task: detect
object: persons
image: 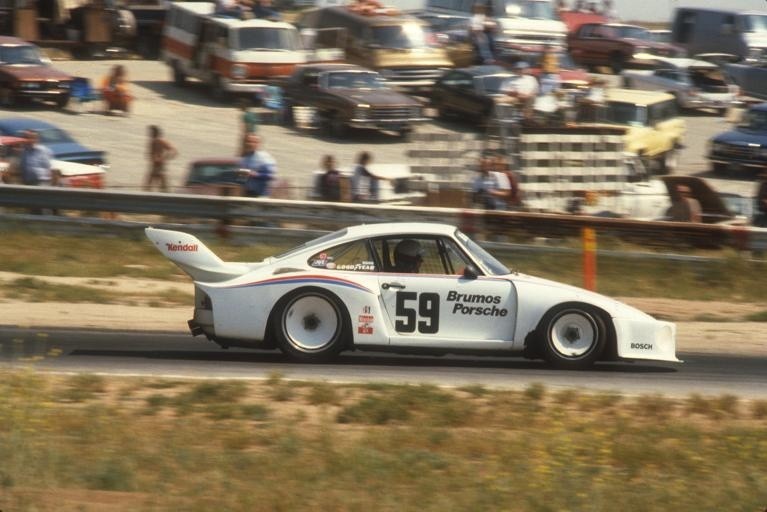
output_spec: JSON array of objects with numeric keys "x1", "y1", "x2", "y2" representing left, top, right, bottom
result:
[
  {"x1": 499, "y1": 55, "x2": 566, "y2": 135},
  {"x1": 113, "y1": 4, "x2": 141, "y2": 61},
  {"x1": 468, "y1": 1, "x2": 497, "y2": 66},
  {"x1": 314, "y1": 152, "x2": 391, "y2": 204},
  {"x1": 660, "y1": 152, "x2": 678, "y2": 196},
  {"x1": 555, "y1": 0, "x2": 617, "y2": 22},
  {"x1": 235, "y1": 107, "x2": 279, "y2": 197},
  {"x1": 392, "y1": 240, "x2": 425, "y2": 272},
  {"x1": 17, "y1": 130, "x2": 62, "y2": 219},
  {"x1": 757, "y1": 181, "x2": 767, "y2": 213},
  {"x1": 146, "y1": 125, "x2": 178, "y2": 191},
  {"x1": 103, "y1": 66, "x2": 131, "y2": 117},
  {"x1": 671, "y1": 186, "x2": 703, "y2": 222},
  {"x1": 473, "y1": 155, "x2": 523, "y2": 212}
]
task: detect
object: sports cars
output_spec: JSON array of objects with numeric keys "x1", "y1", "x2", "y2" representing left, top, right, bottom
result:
[{"x1": 144, "y1": 221, "x2": 684, "y2": 369}]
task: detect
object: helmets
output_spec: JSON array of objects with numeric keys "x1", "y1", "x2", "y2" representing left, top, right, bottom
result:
[{"x1": 393, "y1": 238, "x2": 424, "y2": 265}]
